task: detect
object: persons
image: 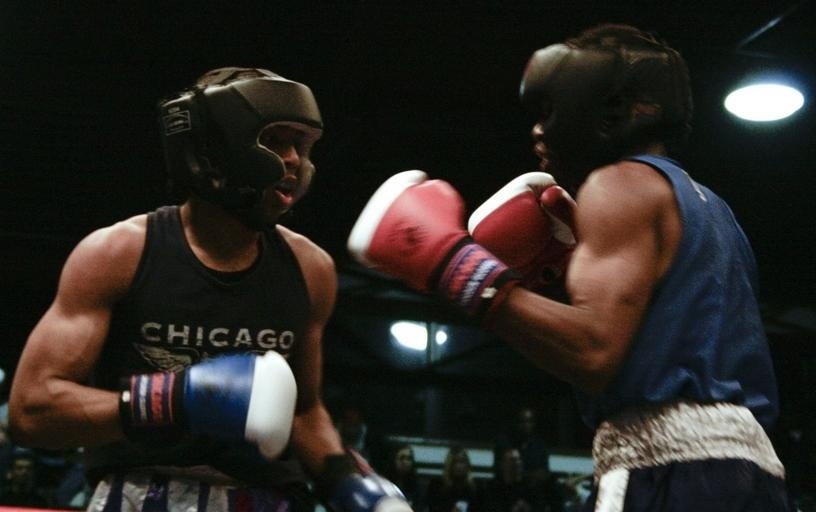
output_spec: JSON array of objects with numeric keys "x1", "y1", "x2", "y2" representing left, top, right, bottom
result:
[
  {"x1": 7, "y1": 65, "x2": 413, "y2": 512},
  {"x1": 345, "y1": 22, "x2": 791, "y2": 512}
]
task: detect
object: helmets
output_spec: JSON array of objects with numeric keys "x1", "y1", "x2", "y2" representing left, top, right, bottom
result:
[
  {"x1": 513, "y1": 22, "x2": 698, "y2": 192},
  {"x1": 152, "y1": 67, "x2": 324, "y2": 208}
]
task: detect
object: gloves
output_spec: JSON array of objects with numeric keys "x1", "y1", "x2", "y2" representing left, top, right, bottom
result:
[
  {"x1": 115, "y1": 348, "x2": 300, "y2": 469},
  {"x1": 347, "y1": 165, "x2": 523, "y2": 324},
  {"x1": 462, "y1": 170, "x2": 581, "y2": 295},
  {"x1": 311, "y1": 449, "x2": 416, "y2": 511}
]
